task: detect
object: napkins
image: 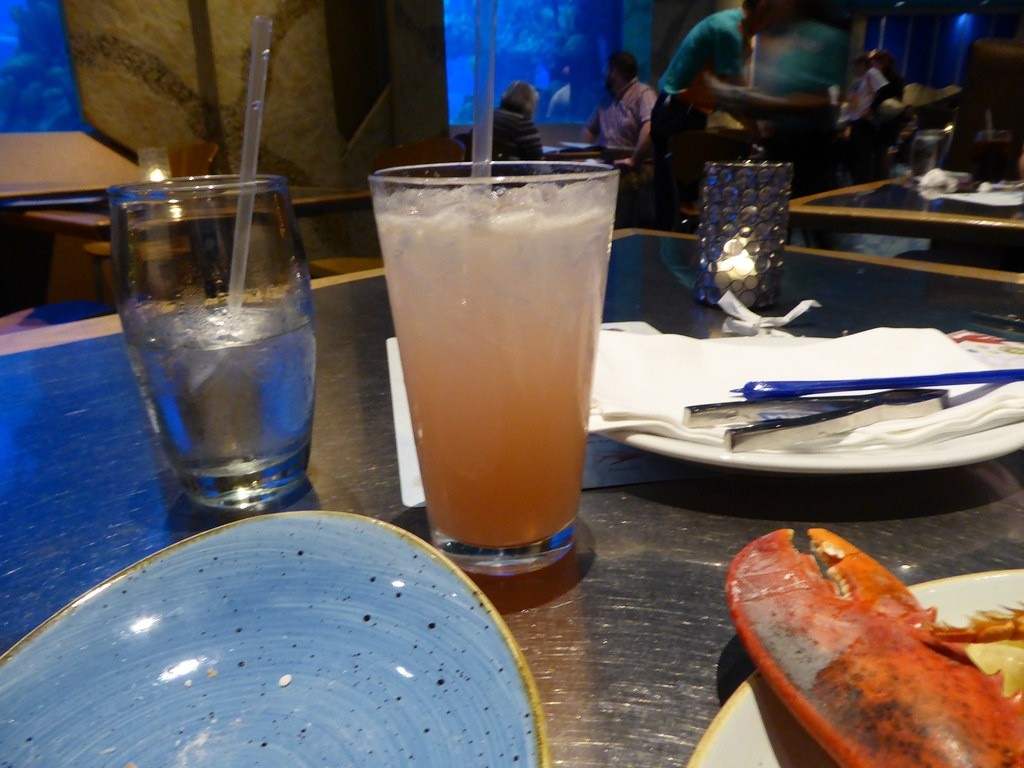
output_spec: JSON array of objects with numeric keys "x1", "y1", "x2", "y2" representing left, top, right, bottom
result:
[{"x1": 589, "y1": 326, "x2": 1024, "y2": 452}]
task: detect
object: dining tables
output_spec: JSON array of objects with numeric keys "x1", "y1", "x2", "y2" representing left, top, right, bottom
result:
[
  {"x1": 1, "y1": 235, "x2": 1024, "y2": 768},
  {"x1": 787, "y1": 180, "x2": 1024, "y2": 245},
  {"x1": 26, "y1": 191, "x2": 372, "y2": 312}
]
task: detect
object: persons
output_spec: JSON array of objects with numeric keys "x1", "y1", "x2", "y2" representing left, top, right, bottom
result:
[
  {"x1": 580, "y1": 53, "x2": 659, "y2": 193},
  {"x1": 462, "y1": 80, "x2": 543, "y2": 189},
  {"x1": 753, "y1": 0, "x2": 852, "y2": 251},
  {"x1": 650, "y1": 0, "x2": 805, "y2": 232},
  {"x1": 832, "y1": 46, "x2": 919, "y2": 186}
]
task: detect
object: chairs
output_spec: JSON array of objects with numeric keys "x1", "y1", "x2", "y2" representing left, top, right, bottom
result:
[{"x1": 84, "y1": 142, "x2": 222, "y2": 307}]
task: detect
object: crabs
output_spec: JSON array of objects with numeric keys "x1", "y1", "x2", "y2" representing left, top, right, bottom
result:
[{"x1": 724, "y1": 526, "x2": 1024, "y2": 768}]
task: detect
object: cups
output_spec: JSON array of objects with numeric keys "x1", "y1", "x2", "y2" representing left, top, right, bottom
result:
[
  {"x1": 694, "y1": 159, "x2": 792, "y2": 312},
  {"x1": 106, "y1": 172, "x2": 318, "y2": 517},
  {"x1": 910, "y1": 128, "x2": 952, "y2": 185},
  {"x1": 369, "y1": 159, "x2": 620, "y2": 577}
]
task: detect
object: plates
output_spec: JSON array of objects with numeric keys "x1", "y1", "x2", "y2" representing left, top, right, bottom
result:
[
  {"x1": 596, "y1": 421, "x2": 1024, "y2": 475},
  {"x1": 0, "y1": 510, "x2": 550, "y2": 768},
  {"x1": 685, "y1": 569, "x2": 1022, "y2": 766}
]
